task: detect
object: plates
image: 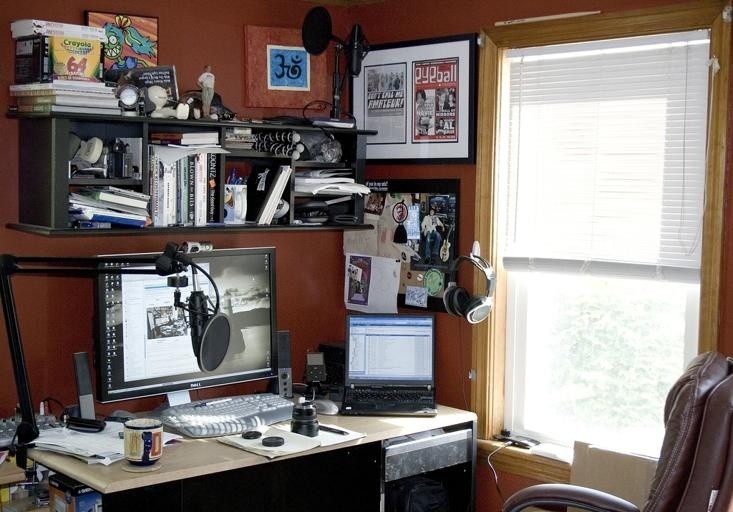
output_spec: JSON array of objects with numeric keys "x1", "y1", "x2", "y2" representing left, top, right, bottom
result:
[{"x1": 424, "y1": 269, "x2": 443, "y2": 297}]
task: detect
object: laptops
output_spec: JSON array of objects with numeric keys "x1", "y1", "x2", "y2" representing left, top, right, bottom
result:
[{"x1": 341, "y1": 314, "x2": 437, "y2": 416}]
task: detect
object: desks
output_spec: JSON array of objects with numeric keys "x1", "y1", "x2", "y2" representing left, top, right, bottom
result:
[{"x1": 25, "y1": 397, "x2": 481, "y2": 510}]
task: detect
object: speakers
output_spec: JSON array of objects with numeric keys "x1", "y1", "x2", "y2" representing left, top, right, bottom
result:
[
  {"x1": 73, "y1": 352, "x2": 96, "y2": 420},
  {"x1": 271, "y1": 330, "x2": 293, "y2": 398}
]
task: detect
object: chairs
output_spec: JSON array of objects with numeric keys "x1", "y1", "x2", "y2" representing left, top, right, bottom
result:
[{"x1": 502, "y1": 345, "x2": 733, "y2": 512}]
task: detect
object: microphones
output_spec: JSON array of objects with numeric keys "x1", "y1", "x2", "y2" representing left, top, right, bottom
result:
[
  {"x1": 188, "y1": 291, "x2": 208, "y2": 357},
  {"x1": 347, "y1": 24, "x2": 364, "y2": 76}
]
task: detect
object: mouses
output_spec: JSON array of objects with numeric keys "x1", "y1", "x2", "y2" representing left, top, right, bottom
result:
[{"x1": 311, "y1": 400, "x2": 339, "y2": 415}]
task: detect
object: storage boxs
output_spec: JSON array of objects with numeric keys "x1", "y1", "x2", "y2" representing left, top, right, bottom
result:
[{"x1": 13, "y1": 36, "x2": 106, "y2": 84}]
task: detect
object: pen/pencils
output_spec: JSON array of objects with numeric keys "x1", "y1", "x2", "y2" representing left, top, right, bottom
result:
[
  {"x1": 106, "y1": 417, "x2": 129, "y2": 422},
  {"x1": 319, "y1": 425, "x2": 348, "y2": 435},
  {"x1": 193, "y1": 397, "x2": 232, "y2": 409}
]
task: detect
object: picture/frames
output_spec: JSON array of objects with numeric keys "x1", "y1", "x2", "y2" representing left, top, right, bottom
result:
[
  {"x1": 86, "y1": 10, "x2": 160, "y2": 80},
  {"x1": 349, "y1": 31, "x2": 479, "y2": 165}
]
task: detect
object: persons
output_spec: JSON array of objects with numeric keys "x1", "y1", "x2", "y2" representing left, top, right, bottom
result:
[
  {"x1": 387, "y1": 71, "x2": 404, "y2": 90},
  {"x1": 420, "y1": 208, "x2": 445, "y2": 265},
  {"x1": 415, "y1": 87, "x2": 456, "y2": 136},
  {"x1": 366, "y1": 195, "x2": 384, "y2": 211},
  {"x1": 395, "y1": 205, "x2": 405, "y2": 220},
  {"x1": 196, "y1": 64, "x2": 215, "y2": 118}
]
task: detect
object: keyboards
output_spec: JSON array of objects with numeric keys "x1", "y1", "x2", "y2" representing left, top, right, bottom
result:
[{"x1": 159, "y1": 393, "x2": 295, "y2": 437}]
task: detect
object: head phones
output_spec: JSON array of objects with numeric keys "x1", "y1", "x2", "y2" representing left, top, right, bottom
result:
[{"x1": 443, "y1": 255, "x2": 496, "y2": 324}]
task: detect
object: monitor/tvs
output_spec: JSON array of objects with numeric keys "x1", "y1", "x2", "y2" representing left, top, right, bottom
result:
[{"x1": 92, "y1": 247, "x2": 279, "y2": 407}]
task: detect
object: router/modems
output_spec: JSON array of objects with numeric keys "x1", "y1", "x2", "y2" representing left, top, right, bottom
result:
[{"x1": 501, "y1": 435, "x2": 540, "y2": 449}]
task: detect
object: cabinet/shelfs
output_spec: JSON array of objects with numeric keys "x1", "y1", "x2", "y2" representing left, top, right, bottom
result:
[{"x1": 5, "y1": 104, "x2": 378, "y2": 240}]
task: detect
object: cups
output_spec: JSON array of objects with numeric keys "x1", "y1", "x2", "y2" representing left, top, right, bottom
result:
[
  {"x1": 225, "y1": 185, "x2": 247, "y2": 225},
  {"x1": 124, "y1": 418, "x2": 163, "y2": 466}
]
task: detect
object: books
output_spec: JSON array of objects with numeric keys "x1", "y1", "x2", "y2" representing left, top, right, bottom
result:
[
  {"x1": 9, "y1": 19, "x2": 123, "y2": 116},
  {"x1": 245, "y1": 164, "x2": 293, "y2": 226},
  {"x1": 67, "y1": 130, "x2": 222, "y2": 230}
]
